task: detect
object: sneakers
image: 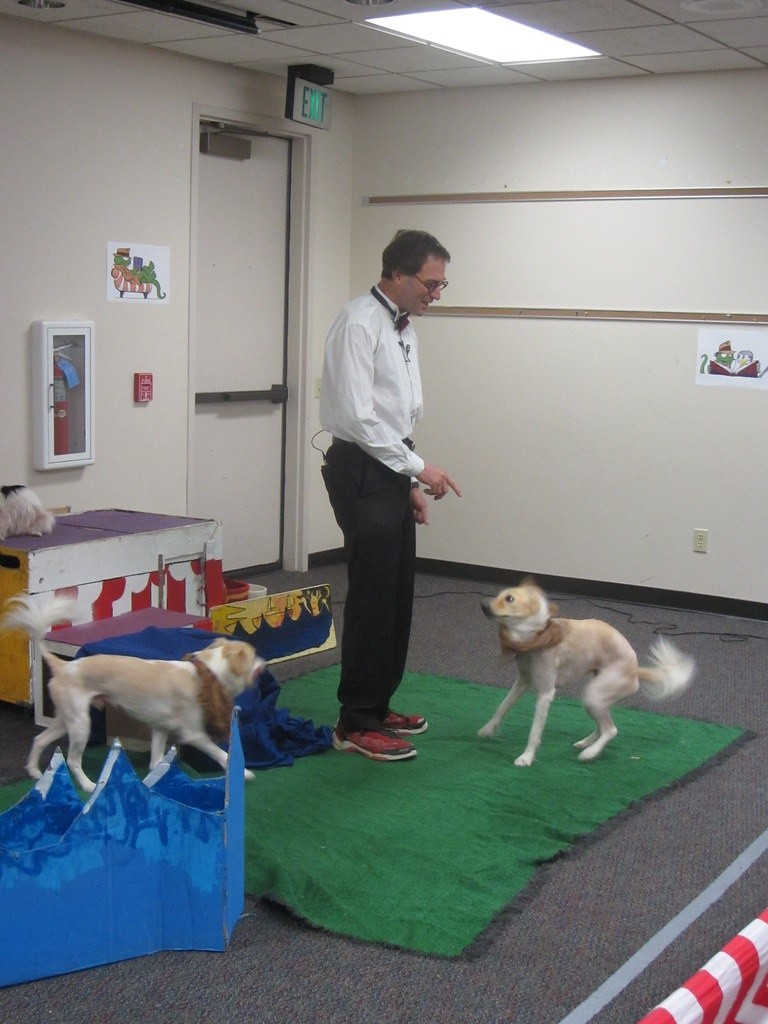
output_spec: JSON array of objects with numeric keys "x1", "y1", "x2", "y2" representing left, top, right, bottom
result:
[
  {"x1": 382, "y1": 708, "x2": 428, "y2": 736},
  {"x1": 332, "y1": 713, "x2": 417, "y2": 761}
]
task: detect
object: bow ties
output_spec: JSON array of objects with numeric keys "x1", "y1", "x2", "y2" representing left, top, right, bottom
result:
[{"x1": 370, "y1": 285, "x2": 411, "y2": 332}]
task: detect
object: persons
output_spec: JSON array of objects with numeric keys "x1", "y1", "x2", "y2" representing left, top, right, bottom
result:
[{"x1": 319, "y1": 229, "x2": 461, "y2": 759}]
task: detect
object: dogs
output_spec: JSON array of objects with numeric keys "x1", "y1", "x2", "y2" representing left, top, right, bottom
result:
[
  {"x1": 0, "y1": 485, "x2": 56, "y2": 542},
  {"x1": 5, "y1": 594, "x2": 267, "y2": 792},
  {"x1": 478, "y1": 581, "x2": 698, "y2": 767}
]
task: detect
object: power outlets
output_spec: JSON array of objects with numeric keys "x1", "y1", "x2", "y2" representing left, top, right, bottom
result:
[{"x1": 693, "y1": 528, "x2": 710, "y2": 554}]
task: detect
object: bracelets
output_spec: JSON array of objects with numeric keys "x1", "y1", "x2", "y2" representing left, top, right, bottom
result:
[{"x1": 411, "y1": 482, "x2": 419, "y2": 489}]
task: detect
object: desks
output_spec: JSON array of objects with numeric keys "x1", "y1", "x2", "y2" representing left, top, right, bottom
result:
[{"x1": 0, "y1": 508, "x2": 221, "y2": 707}]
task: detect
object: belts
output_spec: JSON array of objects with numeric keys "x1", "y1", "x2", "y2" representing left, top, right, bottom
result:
[{"x1": 331, "y1": 434, "x2": 417, "y2": 454}]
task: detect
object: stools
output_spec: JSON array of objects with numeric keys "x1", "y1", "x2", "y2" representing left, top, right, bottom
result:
[{"x1": 30, "y1": 607, "x2": 212, "y2": 728}]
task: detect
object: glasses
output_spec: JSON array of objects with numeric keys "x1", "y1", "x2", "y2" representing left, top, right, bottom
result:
[{"x1": 413, "y1": 271, "x2": 449, "y2": 291}]
